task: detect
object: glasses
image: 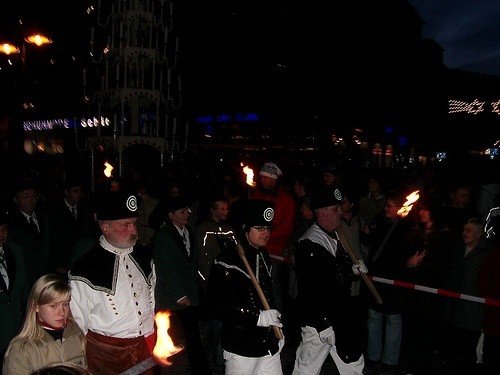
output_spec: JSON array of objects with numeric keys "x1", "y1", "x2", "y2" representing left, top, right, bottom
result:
[{"x1": 251, "y1": 226, "x2": 272, "y2": 232}]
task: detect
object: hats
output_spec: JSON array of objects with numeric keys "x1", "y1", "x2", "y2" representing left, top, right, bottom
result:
[
  {"x1": 259, "y1": 163, "x2": 282, "y2": 180},
  {"x1": 310, "y1": 188, "x2": 343, "y2": 210},
  {"x1": 97, "y1": 193, "x2": 143, "y2": 220},
  {"x1": 351, "y1": 259, "x2": 369, "y2": 275},
  {"x1": 245, "y1": 204, "x2": 274, "y2": 226}
]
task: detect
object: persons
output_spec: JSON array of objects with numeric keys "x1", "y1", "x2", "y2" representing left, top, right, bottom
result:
[
  {"x1": 0, "y1": 272, "x2": 88, "y2": 375},
  {"x1": 0, "y1": 140, "x2": 500, "y2": 375},
  {"x1": 30, "y1": 360, "x2": 95, "y2": 375},
  {"x1": 205, "y1": 196, "x2": 293, "y2": 375},
  {"x1": 61, "y1": 188, "x2": 166, "y2": 374},
  {"x1": 282, "y1": 178, "x2": 373, "y2": 375}
]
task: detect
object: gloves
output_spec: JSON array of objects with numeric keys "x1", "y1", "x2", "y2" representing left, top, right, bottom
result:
[
  {"x1": 319, "y1": 326, "x2": 336, "y2": 346},
  {"x1": 257, "y1": 309, "x2": 283, "y2": 328}
]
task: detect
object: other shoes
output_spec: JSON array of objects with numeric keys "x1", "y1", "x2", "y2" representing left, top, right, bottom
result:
[
  {"x1": 365, "y1": 358, "x2": 380, "y2": 367},
  {"x1": 380, "y1": 363, "x2": 399, "y2": 372}
]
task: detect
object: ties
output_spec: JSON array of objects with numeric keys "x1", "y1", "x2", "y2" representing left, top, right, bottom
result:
[
  {"x1": 29, "y1": 217, "x2": 38, "y2": 232},
  {"x1": 72, "y1": 208, "x2": 75, "y2": 215}
]
